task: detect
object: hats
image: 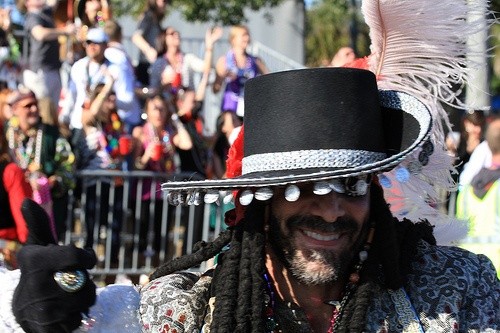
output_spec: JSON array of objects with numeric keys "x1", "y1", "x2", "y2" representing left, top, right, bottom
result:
[
  {"x1": 161, "y1": 0, "x2": 499, "y2": 206},
  {"x1": 7, "y1": 88, "x2": 34, "y2": 104},
  {"x1": 86, "y1": 28, "x2": 108, "y2": 43}
]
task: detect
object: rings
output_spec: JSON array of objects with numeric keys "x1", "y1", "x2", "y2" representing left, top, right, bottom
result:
[{"x1": 52, "y1": 270, "x2": 84, "y2": 305}]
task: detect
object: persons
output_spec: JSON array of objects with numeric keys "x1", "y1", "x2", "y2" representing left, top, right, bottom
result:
[
  {"x1": 0, "y1": 0, "x2": 500, "y2": 288},
  {"x1": 12, "y1": 67, "x2": 500, "y2": 333}
]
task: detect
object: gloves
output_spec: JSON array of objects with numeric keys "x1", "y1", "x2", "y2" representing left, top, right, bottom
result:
[{"x1": 12, "y1": 199, "x2": 97, "y2": 333}]
